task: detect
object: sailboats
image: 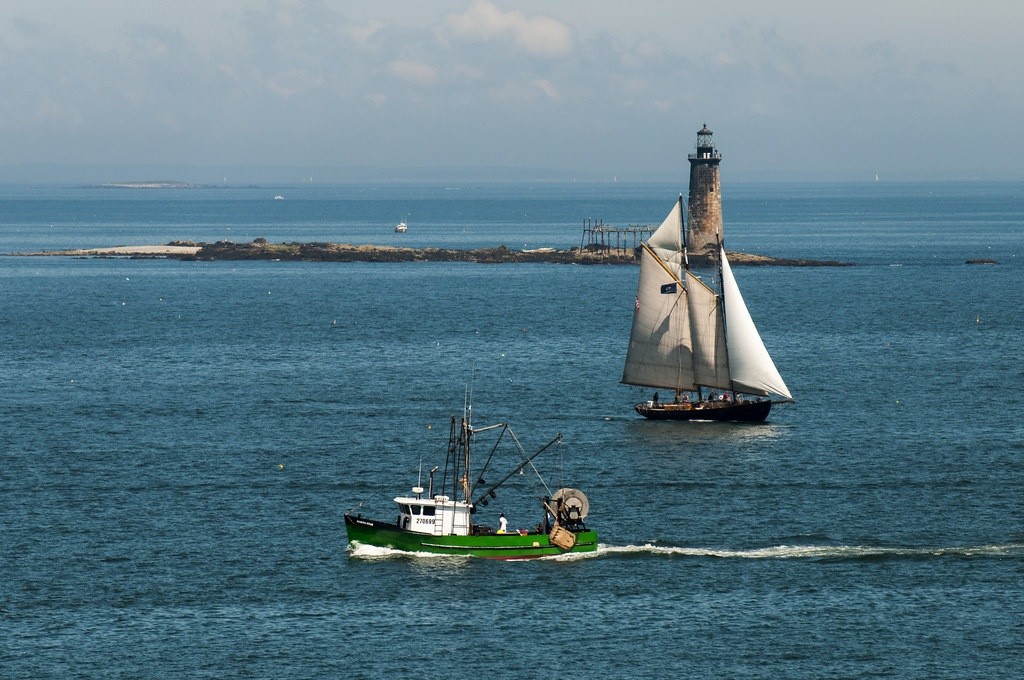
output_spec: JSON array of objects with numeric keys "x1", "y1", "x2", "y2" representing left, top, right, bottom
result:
[{"x1": 618, "y1": 193, "x2": 797, "y2": 425}]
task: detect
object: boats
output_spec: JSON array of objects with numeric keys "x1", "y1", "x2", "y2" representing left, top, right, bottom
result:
[
  {"x1": 395, "y1": 223, "x2": 408, "y2": 234},
  {"x1": 345, "y1": 381, "x2": 600, "y2": 557}
]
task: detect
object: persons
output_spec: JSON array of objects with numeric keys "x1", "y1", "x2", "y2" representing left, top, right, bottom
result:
[
  {"x1": 499, "y1": 513, "x2": 508, "y2": 533},
  {"x1": 653, "y1": 391, "x2": 659, "y2": 405},
  {"x1": 683, "y1": 394, "x2": 687, "y2": 401},
  {"x1": 708, "y1": 392, "x2": 714, "y2": 402},
  {"x1": 723, "y1": 391, "x2": 729, "y2": 399},
  {"x1": 674, "y1": 397, "x2": 679, "y2": 404}
]
